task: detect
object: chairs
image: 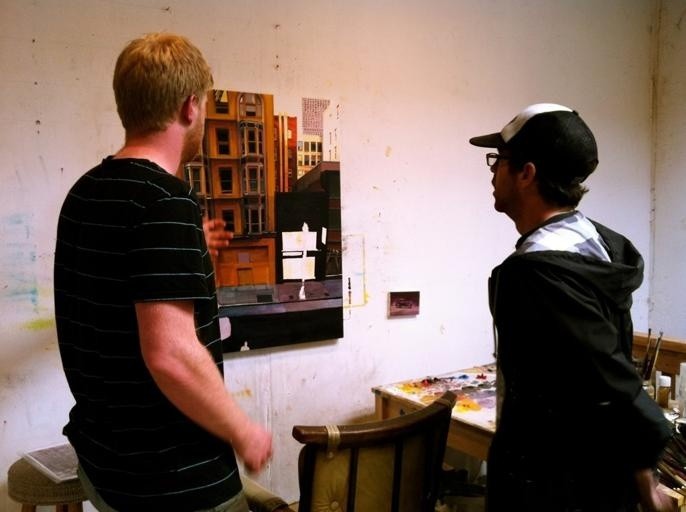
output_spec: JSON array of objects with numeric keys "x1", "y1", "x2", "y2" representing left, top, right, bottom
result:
[{"x1": 240, "y1": 389, "x2": 458, "y2": 511}]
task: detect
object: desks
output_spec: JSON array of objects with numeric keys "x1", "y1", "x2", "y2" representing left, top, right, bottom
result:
[{"x1": 369, "y1": 330, "x2": 686, "y2": 507}]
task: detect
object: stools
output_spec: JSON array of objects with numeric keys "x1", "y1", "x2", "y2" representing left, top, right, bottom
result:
[{"x1": 6, "y1": 443, "x2": 91, "y2": 511}]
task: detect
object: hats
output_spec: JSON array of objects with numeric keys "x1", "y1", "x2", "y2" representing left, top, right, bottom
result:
[{"x1": 469, "y1": 103, "x2": 598, "y2": 185}]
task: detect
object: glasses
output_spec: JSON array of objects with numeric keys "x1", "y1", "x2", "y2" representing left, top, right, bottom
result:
[{"x1": 486, "y1": 152, "x2": 514, "y2": 166}]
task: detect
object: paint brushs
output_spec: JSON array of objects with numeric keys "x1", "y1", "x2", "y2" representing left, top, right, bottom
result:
[
  {"x1": 656, "y1": 431, "x2": 686, "y2": 497},
  {"x1": 639, "y1": 328, "x2": 663, "y2": 380}
]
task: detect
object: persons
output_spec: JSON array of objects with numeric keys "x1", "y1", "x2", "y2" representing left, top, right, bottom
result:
[
  {"x1": 470, "y1": 103, "x2": 675, "y2": 512},
  {"x1": 55, "y1": 34, "x2": 276, "y2": 511}
]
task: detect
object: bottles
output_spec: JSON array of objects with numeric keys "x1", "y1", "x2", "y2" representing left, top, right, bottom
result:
[
  {"x1": 657, "y1": 376, "x2": 672, "y2": 410},
  {"x1": 642, "y1": 380, "x2": 654, "y2": 400},
  {"x1": 679, "y1": 362, "x2": 686, "y2": 418}
]
